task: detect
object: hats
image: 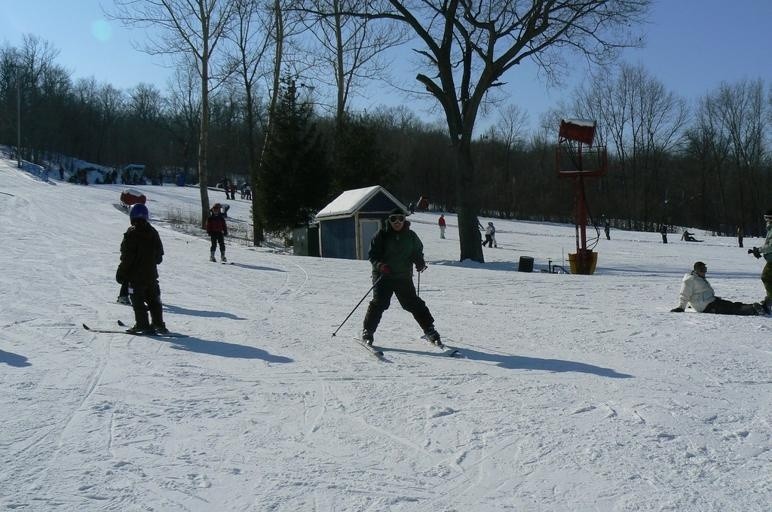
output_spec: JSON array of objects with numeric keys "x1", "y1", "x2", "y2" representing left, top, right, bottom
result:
[
  {"x1": 694, "y1": 261, "x2": 706, "y2": 271},
  {"x1": 389, "y1": 207, "x2": 404, "y2": 215}
]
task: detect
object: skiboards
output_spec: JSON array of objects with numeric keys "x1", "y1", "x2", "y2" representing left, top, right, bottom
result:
[
  {"x1": 82, "y1": 321, "x2": 191, "y2": 337},
  {"x1": 107, "y1": 297, "x2": 151, "y2": 313},
  {"x1": 353, "y1": 333, "x2": 459, "y2": 357},
  {"x1": 209, "y1": 259, "x2": 234, "y2": 265}
]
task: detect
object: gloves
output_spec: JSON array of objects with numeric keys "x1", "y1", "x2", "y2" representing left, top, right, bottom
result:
[
  {"x1": 670, "y1": 305, "x2": 692, "y2": 313},
  {"x1": 748, "y1": 246, "x2": 762, "y2": 259},
  {"x1": 377, "y1": 263, "x2": 390, "y2": 275},
  {"x1": 416, "y1": 263, "x2": 428, "y2": 273}
]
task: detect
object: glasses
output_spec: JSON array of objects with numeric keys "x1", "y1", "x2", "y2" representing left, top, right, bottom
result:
[
  {"x1": 389, "y1": 216, "x2": 405, "y2": 224},
  {"x1": 764, "y1": 215, "x2": 772, "y2": 221}
]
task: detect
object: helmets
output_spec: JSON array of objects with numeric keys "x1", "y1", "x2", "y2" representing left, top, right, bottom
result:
[{"x1": 130, "y1": 203, "x2": 149, "y2": 220}]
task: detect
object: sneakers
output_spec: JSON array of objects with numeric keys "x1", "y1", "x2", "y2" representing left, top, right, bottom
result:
[
  {"x1": 426, "y1": 331, "x2": 441, "y2": 346},
  {"x1": 126, "y1": 321, "x2": 166, "y2": 332},
  {"x1": 753, "y1": 295, "x2": 772, "y2": 315},
  {"x1": 210, "y1": 256, "x2": 227, "y2": 262},
  {"x1": 361, "y1": 330, "x2": 375, "y2": 346},
  {"x1": 117, "y1": 296, "x2": 130, "y2": 305}
]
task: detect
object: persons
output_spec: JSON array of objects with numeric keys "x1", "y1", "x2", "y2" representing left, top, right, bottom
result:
[
  {"x1": 489, "y1": 222, "x2": 497, "y2": 247},
  {"x1": 733, "y1": 223, "x2": 743, "y2": 247},
  {"x1": 56, "y1": 164, "x2": 252, "y2": 201},
  {"x1": 120, "y1": 203, "x2": 169, "y2": 334},
  {"x1": 438, "y1": 213, "x2": 446, "y2": 240},
  {"x1": 116, "y1": 279, "x2": 149, "y2": 311},
  {"x1": 206, "y1": 205, "x2": 230, "y2": 263},
  {"x1": 481, "y1": 222, "x2": 492, "y2": 248},
  {"x1": 602, "y1": 217, "x2": 610, "y2": 240},
  {"x1": 679, "y1": 230, "x2": 696, "y2": 241},
  {"x1": 747, "y1": 208, "x2": 771, "y2": 314},
  {"x1": 358, "y1": 208, "x2": 442, "y2": 348},
  {"x1": 659, "y1": 225, "x2": 667, "y2": 243},
  {"x1": 669, "y1": 262, "x2": 769, "y2": 316}
]
